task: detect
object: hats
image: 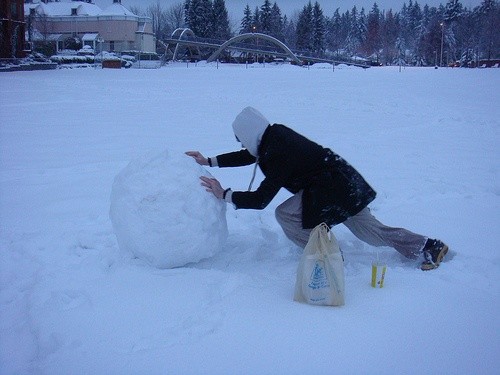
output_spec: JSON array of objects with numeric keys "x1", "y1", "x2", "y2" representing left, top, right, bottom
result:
[{"x1": 231, "y1": 106, "x2": 270, "y2": 158}]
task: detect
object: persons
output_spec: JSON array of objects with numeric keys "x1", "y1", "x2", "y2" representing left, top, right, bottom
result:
[{"x1": 186, "y1": 107, "x2": 448, "y2": 270}]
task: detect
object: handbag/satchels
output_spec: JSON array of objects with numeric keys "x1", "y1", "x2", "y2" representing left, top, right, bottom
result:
[{"x1": 292, "y1": 222, "x2": 346, "y2": 306}]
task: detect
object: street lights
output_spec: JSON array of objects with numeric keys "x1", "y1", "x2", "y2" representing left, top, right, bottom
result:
[
  {"x1": 440, "y1": 23, "x2": 444, "y2": 67},
  {"x1": 253, "y1": 26, "x2": 259, "y2": 62}
]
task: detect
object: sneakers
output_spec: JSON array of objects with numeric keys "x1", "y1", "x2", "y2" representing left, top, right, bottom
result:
[{"x1": 420, "y1": 239, "x2": 449, "y2": 270}]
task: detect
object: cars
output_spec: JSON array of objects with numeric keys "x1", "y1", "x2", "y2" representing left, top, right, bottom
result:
[{"x1": 179, "y1": 55, "x2": 201, "y2": 63}]
